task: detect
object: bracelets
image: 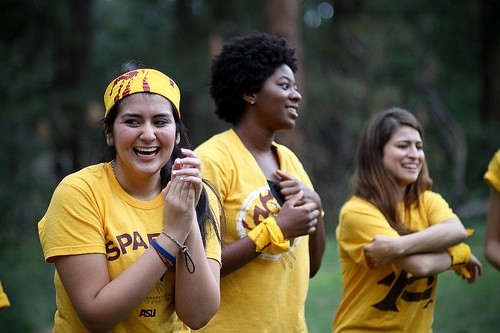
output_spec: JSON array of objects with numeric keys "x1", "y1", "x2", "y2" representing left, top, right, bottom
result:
[
  {"x1": 149, "y1": 238, "x2": 176, "y2": 270},
  {"x1": 161, "y1": 231, "x2": 195, "y2": 274}
]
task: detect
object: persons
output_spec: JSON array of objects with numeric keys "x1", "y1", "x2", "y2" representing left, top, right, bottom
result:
[
  {"x1": 37, "y1": 69, "x2": 227, "y2": 333},
  {"x1": 192, "y1": 31, "x2": 327, "y2": 333},
  {"x1": 335, "y1": 106, "x2": 482, "y2": 333},
  {"x1": 483, "y1": 148, "x2": 500, "y2": 272}
]
